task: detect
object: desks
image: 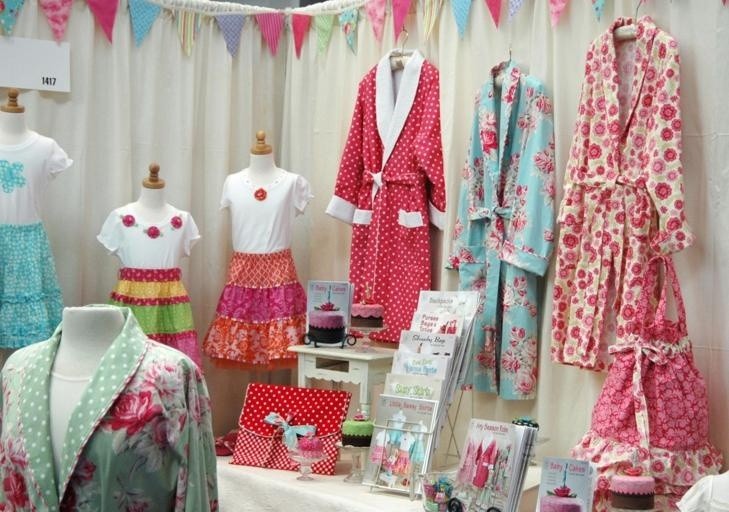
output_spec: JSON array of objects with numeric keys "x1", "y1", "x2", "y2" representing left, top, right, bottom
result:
[
  {"x1": 289, "y1": 344, "x2": 396, "y2": 419},
  {"x1": 216, "y1": 445, "x2": 543, "y2": 511}
]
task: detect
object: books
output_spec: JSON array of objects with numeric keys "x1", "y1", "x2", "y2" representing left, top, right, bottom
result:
[
  {"x1": 362, "y1": 288, "x2": 486, "y2": 498},
  {"x1": 453, "y1": 416, "x2": 537, "y2": 512},
  {"x1": 536, "y1": 455, "x2": 598, "y2": 512},
  {"x1": 305, "y1": 279, "x2": 353, "y2": 336}
]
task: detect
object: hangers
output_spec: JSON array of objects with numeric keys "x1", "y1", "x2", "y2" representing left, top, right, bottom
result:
[
  {"x1": 493, "y1": 42, "x2": 511, "y2": 89},
  {"x1": 388, "y1": 27, "x2": 409, "y2": 72},
  {"x1": 612, "y1": 0, "x2": 642, "y2": 42}
]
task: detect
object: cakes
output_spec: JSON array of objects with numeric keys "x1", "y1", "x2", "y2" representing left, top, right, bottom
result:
[
  {"x1": 341, "y1": 419, "x2": 375, "y2": 448},
  {"x1": 308, "y1": 310, "x2": 345, "y2": 344},
  {"x1": 349, "y1": 302, "x2": 385, "y2": 326},
  {"x1": 539, "y1": 493, "x2": 581, "y2": 511},
  {"x1": 610, "y1": 474, "x2": 656, "y2": 510}
]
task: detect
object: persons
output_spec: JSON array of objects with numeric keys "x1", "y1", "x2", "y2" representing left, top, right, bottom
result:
[
  {"x1": 93, "y1": 177, "x2": 207, "y2": 381},
  {"x1": 201, "y1": 145, "x2": 315, "y2": 370},
  {"x1": 1, "y1": 303, "x2": 220, "y2": 512},
  {"x1": 1, "y1": 104, "x2": 76, "y2": 352}
]
